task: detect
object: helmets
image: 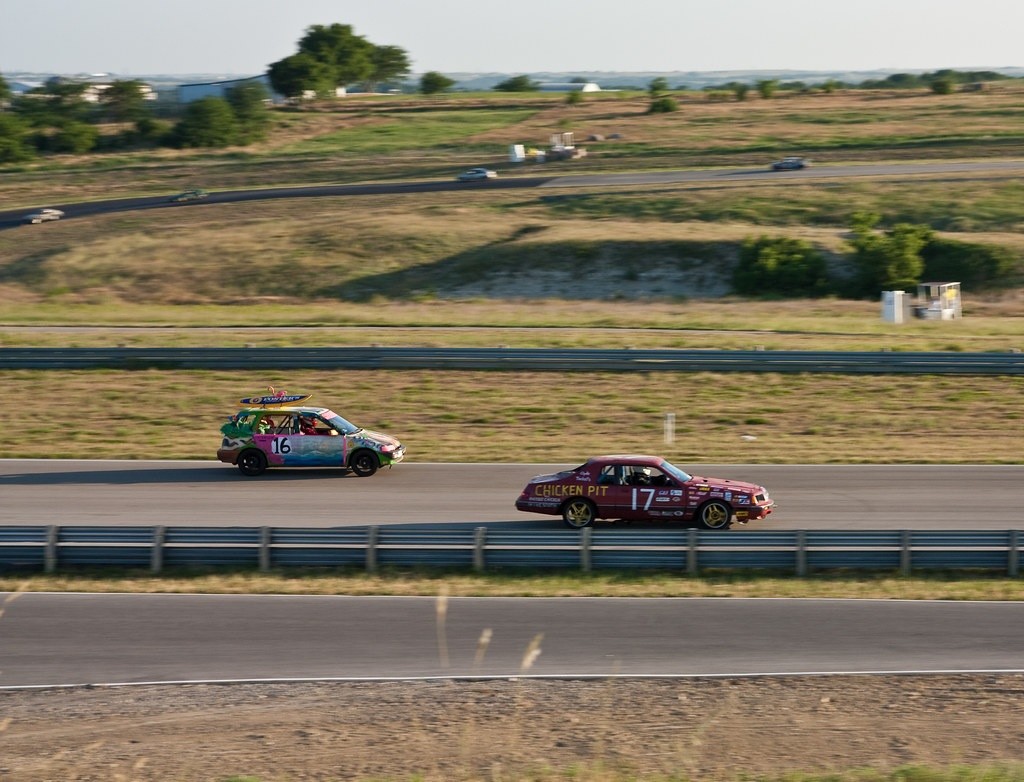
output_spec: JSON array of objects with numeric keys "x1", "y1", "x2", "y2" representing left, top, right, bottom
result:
[
  {"x1": 633, "y1": 466, "x2": 651, "y2": 475},
  {"x1": 298, "y1": 415, "x2": 317, "y2": 426}
]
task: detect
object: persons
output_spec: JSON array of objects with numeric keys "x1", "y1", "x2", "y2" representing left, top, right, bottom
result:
[
  {"x1": 301, "y1": 415, "x2": 339, "y2": 437},
  {"x1": 631, "y1": 466, "x2": 671, "y2": 487}
]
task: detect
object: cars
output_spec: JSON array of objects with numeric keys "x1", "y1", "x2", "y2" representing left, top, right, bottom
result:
[
  {"x1": 171, "y1": 190, "x2": 209, "y2": 205},
  {"x1": 772, "y1": 156, "x2": 803, "y2": 173},
  {"x1": 20, "y1": 207, "x2": 65, "y2": 226},
  {"x1": 512, "y1": 454, "x2": 773, "y2": 531},
  {"x1": 458, "y1": 168, "x2": 497, "y2": 182},
  {"x1": 218, "y1": 386, "x2": 407, "y2": 481}
]
